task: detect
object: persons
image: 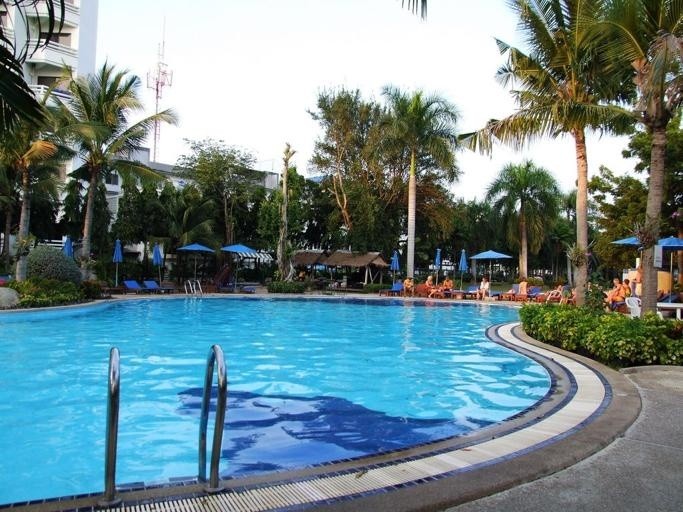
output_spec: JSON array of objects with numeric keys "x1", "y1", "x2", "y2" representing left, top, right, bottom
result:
[
  {"x1": 557, "y1": 285, "x2": 572, "y2": 306},
  {"x1": 297, "y1": 270, "x2": 305, "y2": 282},
  {"x1": 475, "y1": 276, "x2": 489, "y2": 301},
  {"x1": 401, "y1": 276, "x2": 415, "y2": 298},
  {"x1": 435, "y1": 282, "x2": 445, "y2": 299},
  {"x1": 604, "y1": 278, "x2": 625, "y2": 304},
  {"x1": 604, "y1": 278, "x2": 631, "y2": 313},
  {"x1": 423, "y1": 275, "x2": 438, "y2": 298},
  {"x1": 630, "y1": 265, "x2": 642, "y2": 300},
  {"x1": 544, "y1": 285, "x2": 563, "y2": 304},
  {"x1": 441, "y1": 275, "x2": 454, "y2": 299}
]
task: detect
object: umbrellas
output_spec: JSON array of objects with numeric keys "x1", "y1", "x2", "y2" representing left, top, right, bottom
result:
[
  {"x1": 112, "y1": 237, "x2": 122, "y2": 287},
  {"x1": 220, "y1": 243, "x2": 257, "y2": 288},
  {"x1": 62, "y1": 235, "x2": 72, "y2": 260},
  {"x1": 433, "y1": 248, "x2": 441, "y2": 286},
  {"x1": 457, "y1": 249, "x2": 467, "y2": 291},
  {"x1": 608, "y1": 233, "x2": 645, "y2": 269},
  {"x1": 174, "y1": 242, "x2": 214, "y2": 281},
  {"x1": 468, "y1": 250, "x2": 512, "y2": 291},
  {"x1": 389, "y1": 251, "x2": 400, "y2": 287},
  {"x1": 654, "y1": 235, "x2": 683, "y2": 303},
  {"x1": 152, "y1": 242, "x2": 162, "y2": 288}
]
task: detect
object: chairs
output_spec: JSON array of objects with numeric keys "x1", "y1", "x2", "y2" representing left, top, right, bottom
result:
[
  {"x1": 611, "y1": 289, "x2": 679, "y2": 320},
  {"x1": 380, "y1": 282, "x2": 577, "y2": 305},
  {"x1": 123, "y1": 278, "x2": 175, "y2": 295}
]
